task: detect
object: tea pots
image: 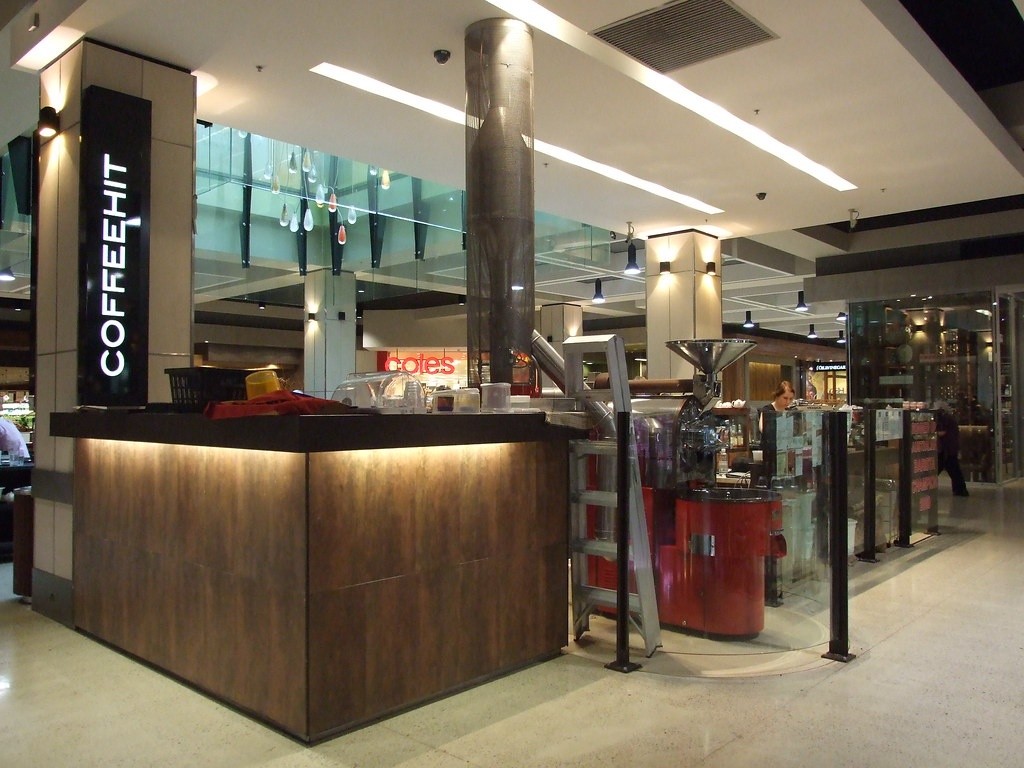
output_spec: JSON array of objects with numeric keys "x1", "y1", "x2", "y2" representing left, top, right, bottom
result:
[{"x1": 732, "y1": 399, "x2": 746, "y2": 408}]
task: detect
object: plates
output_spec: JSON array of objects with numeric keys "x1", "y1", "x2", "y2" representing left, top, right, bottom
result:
[{"x1": 2, "y1": 460, "x2": 10, "y2": 465}]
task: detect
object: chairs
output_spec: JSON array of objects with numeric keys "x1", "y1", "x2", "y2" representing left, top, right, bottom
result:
[{"x1": 957, "y1": 425, "x2": 993, "y2": 482}]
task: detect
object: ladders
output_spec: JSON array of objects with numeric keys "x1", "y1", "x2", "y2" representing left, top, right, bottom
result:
[{"x1": 562, "y1": 334, "x2": 665, "y2": 657}]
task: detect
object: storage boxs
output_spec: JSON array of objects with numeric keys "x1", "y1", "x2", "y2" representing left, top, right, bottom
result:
[{"x1": 164, "y1": 367, "x2": 296, "y2": 411}]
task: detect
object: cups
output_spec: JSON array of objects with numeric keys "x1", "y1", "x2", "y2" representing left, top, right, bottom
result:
[
  {"x1": 454, "y1": 388, "x2": 480, "y2": 413},
  {"x1": 507, "y1": 395, "x2": 530, "y2": 412},
  {"x1": 9, "y1": 450, "x2": 24, "y2": 467},
  {"x1": 0, "y1": 451, "x2": 2, "y2": 465},
  {"x1": 480, "y1": 383, "x2": 511, "y2": 413},
  {"x1": 713, "y1": 400, "x2": 732, "y2": 408},
  {"x1": 752, "y1": 451, "x2": 763, "y2": 461},
  {"x1": 432, "y1": 390, "x2": 455, "y2": 412}
]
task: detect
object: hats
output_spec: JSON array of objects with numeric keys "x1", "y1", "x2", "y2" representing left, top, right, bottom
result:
[{"x1": 929, "y1": 402, "x2": 947, "y2": 410}]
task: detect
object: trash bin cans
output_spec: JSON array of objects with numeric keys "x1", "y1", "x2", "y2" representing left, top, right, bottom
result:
[{"x1": 12, "y1": 485, "x2": 34, "y2": 597}]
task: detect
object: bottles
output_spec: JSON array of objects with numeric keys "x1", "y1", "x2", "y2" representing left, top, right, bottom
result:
[
  {"x1": 719, "y1": 449, "x2": 728, "y2": 476},
  {"x1": 704, "y1": 420, "x2": 743, "y2": 447}
]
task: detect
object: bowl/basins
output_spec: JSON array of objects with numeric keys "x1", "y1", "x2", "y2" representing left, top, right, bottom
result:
[{"x1": 246, "y1": 371, "x2": 281, "y2": 400}]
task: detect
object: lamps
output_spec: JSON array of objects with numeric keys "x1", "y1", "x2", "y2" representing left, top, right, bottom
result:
[
  {"x1": 358, "y1": 287, "x2": 364, "y2": 293},
  {"x1": 356, "y1": 305, "x2": 363, "y2": 319},
  {"x1": 339, "y1": 312, "x2": 345, "y2": 320},
  {"x1": 624, "y1": 222, "x2": 640, "y2": 275},
  {"x1": 592, "y1": 278, "x2": 605, "y2": 304},
  {"x1": 836, "y1": 330, "x2": 846, "y2": 344},
  {"x1": 609, "y1": 231, "x2": 616, "y2": 239},
  {"x1": 807, "y1": 324, "x2": 817, "y2": 338},
  {"x1": 547, "y1": 335, "x2": 552, "y2": 342},
  {"x1": 743, "y1": 311, "x2": 755, "y2": 328},
  {"x1": 795, "y1": 291, "x2": 808, "y2": 312},
  {"x1": 37, "y1": 106, "x2": 60, "y2": 137},
  {"x1": 258, "y1": 302, "x2": 265, "y2": 310},
  {"x1": 263, "y1": 130, "x2": 357, "y2": 245},
  {"x1": 370, "y1": 165, "x2": 390, "y2": 189},
  {"x1": 308, "y1": 313, "x2": 315, "y2": 320},
  {"x1": 850, "y1": 209, "x2": 859, "y2": 227},
  {"x1": 835, "y1": 312, "x2": 846, "y2": 320},
  {"x1": 707, "y1": 261, "x2": 715, "y2": 275},
  {"x1": 659, "y1": 262, "x2": 670, "y2": 275}
]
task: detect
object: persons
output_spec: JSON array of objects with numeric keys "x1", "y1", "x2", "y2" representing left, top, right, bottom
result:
[
  {"x1": 0, "y1": 415, "x2": 32, "y2": 496},
  {"x1": 970, "y1": 395, "x2": 980, "y2": 426},
  {"x1": 932, "y1": 398, "x2": 970, "y2": 498},
  {"x1": 755, "y1": 379, "x2": 797, "y2": 490}
]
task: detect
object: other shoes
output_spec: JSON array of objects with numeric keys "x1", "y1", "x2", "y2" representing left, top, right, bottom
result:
[{"x1": 953, "y1": 488, "x2": 968, "y2": 496}]
